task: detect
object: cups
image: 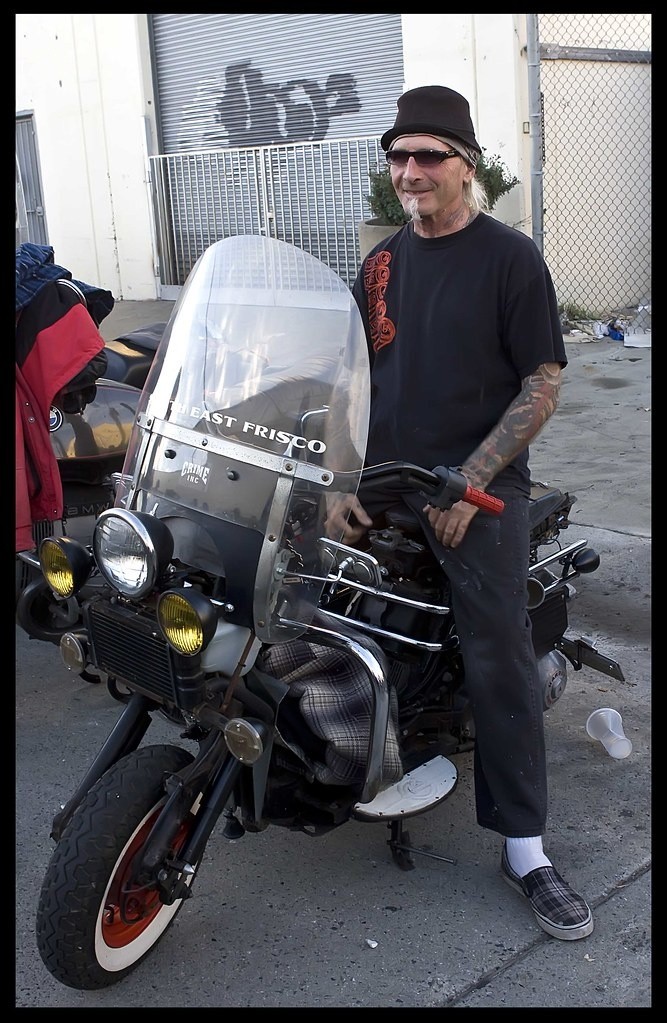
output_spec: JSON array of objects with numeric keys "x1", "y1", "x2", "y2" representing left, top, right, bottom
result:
[{"x1": 585, "y1": 707, "x2": 633, "y2": 759}]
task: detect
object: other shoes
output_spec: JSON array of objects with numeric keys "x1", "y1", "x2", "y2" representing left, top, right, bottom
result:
[{"x1": 502, "y1": 840, "x2": 595, "y2": 940}]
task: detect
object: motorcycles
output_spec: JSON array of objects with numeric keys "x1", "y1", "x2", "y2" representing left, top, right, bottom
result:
[
  {"x1": 18, "y1": 233, "x2": 626, "y2": 992},
  {"x1": 15, "y1": 243, "x2": 343, "y2": 550}
]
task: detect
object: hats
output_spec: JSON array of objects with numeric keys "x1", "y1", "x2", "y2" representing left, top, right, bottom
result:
[{"x1": 381, "y1": 86, "x2": 482, "y2": 152}]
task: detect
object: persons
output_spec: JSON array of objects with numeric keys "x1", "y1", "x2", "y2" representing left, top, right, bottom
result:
[{"x1": 322, "y1": 86, "x2": 597, "y2": 940}]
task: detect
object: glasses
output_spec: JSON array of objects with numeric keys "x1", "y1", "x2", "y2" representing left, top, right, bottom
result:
[{"x1": 383, "y1": 148, "x2": 457, "y2": 166}]
